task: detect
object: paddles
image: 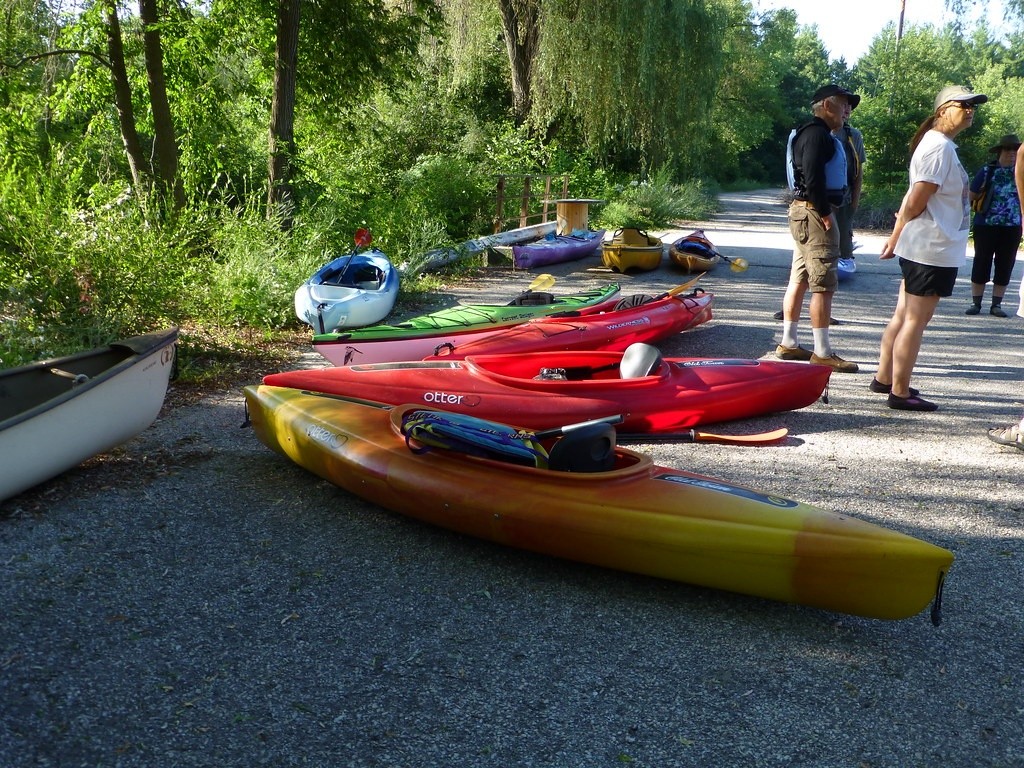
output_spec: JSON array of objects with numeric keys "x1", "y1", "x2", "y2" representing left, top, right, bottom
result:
[
  {"x1": 616, "y1": 428, "x2": 789, "y2": 444},
  {"x1": 644, "y1": 271, "x2": 706, "y2": 304},
  {"x1": 711, "y1": 249, "x2": 748, "y2": 272},
  {"x1": 507, "y1": 273, "x2": 556, "y2": 306},
  {"x1": 338, "y1": 228, "x2": 372, "y2": 285}
]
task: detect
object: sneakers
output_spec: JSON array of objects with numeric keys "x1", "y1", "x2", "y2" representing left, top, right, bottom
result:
[
  {"x1": 869, "y1": 376, "x2": 919, "y2": 395},
  {"x1": 887, "y1": 390, "x2": 939, "y2": 411},
  {"x1": 809, "y1": 351, "x2": 859, "y2": 373},
  {"x1": 775, "y1": 344, "x2": 812, "y2": 360}
]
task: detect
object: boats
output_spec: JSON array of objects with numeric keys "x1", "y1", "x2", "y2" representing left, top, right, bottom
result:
[
  {"x1": 0, "y1": 326, "x2": 180, "y2": 500},
  {"x1": 493, "y1": 229, "x2": 605, "y2": 269},
  {"x1": 262, "y1": 343, "x2": 833, "y2": 435},
  {"x1": 294, "y1": 229, "x2": 399, "y2": 334},
  {"x1": 597, "y1": 228, "x2": 664, "y2": 275},
  {"x1": 668, "y1": 228, "x2": 720, "y2": 273},
  {"x1": 241, "y1": 386, "x2": 955, "y2": 628},
  {"x1": 310, "y1": 269, "x2": 713, "y2": 367}
]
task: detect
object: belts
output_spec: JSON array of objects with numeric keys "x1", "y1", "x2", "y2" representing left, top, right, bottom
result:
[{"x1": 795, "y1": 201, "x2": 812, "y2": 207}]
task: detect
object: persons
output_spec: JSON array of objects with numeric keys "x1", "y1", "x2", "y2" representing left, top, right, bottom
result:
[
  {"x1": 987, "y1": 140, "x2": 1024, "y2": 451},
  {"x1": 965, "y1": 135, "x2": 1022, "y2": 318},
  {"x1": 774, "y1": 85, "x2": 862, "y2": 373},
  {"x1": 869, "y1": 85, "x2": 978, "y2": 410}
]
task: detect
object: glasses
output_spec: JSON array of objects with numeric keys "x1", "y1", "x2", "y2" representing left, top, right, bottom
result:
[
  {"x1": 837, "y1": 88, "x2": 849, "y2": 94},
  {"x1": 946, "y1": 102, "x2": 979, "y2": 110}
]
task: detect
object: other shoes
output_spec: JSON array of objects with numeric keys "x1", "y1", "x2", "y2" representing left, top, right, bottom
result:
[
  {"x1": 990, "y1": 304, "x2": 1007, "y2": 317},
  {"x1": 829, "y1": 318, "x2": 838, "y2": 325},
  {"x1": 773, "y1": 310, "x2": 783, "y2": 319},
  {"x1": 966, "y1": 305, "x2": 980, "y2": 315}
]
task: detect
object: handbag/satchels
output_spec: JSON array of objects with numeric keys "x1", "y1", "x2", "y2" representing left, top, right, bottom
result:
[{"x1": 972, "y1": 163, "x2": 995, "y2": 213}]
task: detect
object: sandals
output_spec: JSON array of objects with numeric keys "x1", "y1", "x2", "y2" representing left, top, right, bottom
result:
[{"x1": 987, "y1": 425, "x2": 1024, "y2": 450}]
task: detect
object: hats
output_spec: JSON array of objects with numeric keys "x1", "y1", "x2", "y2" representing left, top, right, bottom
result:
[
  {"x1": 810, "y1": 85, "x2": 860, "y2": 110},
  {"x1": 988, "y1": 135, "x2": 1023, "y2": 153},
  {"x1": 933, "y1": 86, "x2": 988, "y2": 113}
]
